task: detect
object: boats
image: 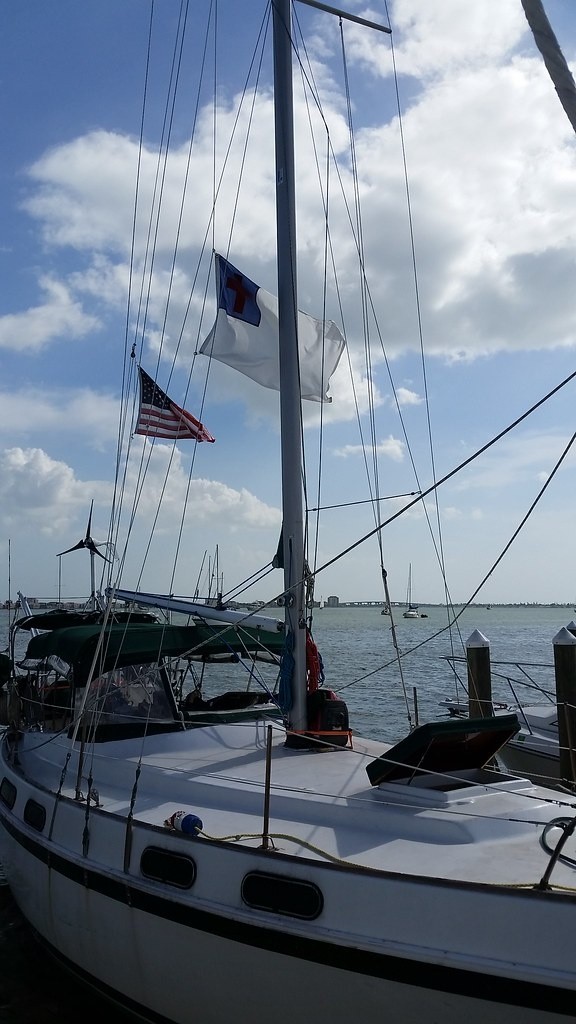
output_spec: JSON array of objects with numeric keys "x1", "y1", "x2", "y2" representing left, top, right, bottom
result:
[{"x1": 381, "y1": 603, "x2": 391, "y2": 615}]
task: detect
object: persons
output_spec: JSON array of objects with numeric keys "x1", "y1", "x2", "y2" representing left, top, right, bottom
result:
[{"x1": 0, "y1": 655, "x2": 11, "y2": 728}]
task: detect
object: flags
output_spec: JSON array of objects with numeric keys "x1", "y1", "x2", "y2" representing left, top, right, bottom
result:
[
  {"x1": 201, "y1": 253, "x2": 346, "y2": 404},
  {"x1": 135, "y1": 367, "x2": 215, "y2": 443}
]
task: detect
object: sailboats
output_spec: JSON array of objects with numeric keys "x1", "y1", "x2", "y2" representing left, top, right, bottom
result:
[
  {"x1": 403, "y1": 560, "x2": 418, "y2": 618},
  {"x1": 1, "y1": 0, "x2": 575, "y2": 1024}
]
